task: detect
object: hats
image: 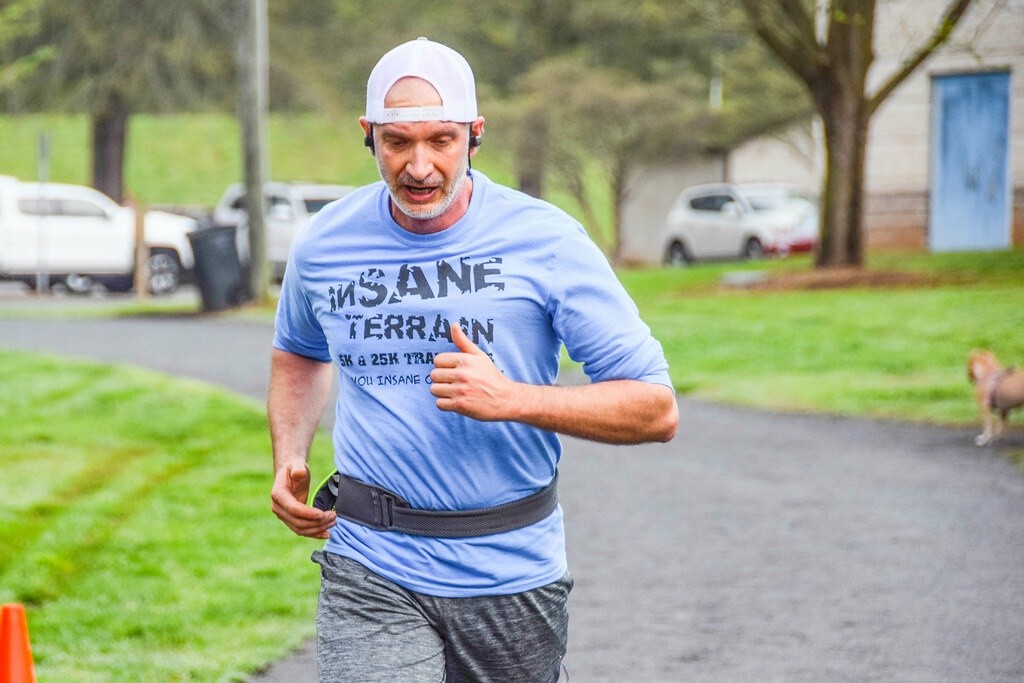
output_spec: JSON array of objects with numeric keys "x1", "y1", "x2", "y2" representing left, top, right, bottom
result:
[{"x1": 366, "y1": 35, "x2": 479, "y2": 124}]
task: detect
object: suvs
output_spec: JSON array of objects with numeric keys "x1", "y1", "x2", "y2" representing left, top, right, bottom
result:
[
  {"x1": 658, "y1": 181, "x2": 824, "y2": 266},
  {"x1": 214, "y1": 178, "x2": 362, "y2": 283},
  {"x1": 0, "y1": 181, "x2": 204, "y2": 300}
]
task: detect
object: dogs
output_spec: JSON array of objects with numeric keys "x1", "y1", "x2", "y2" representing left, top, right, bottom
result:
[{"x1": 966, "y1": 348, "x2": 1023, "y2": 447}]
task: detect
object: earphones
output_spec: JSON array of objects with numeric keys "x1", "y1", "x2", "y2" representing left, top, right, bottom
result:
[
  {"x1": 469, "y1": 121, "x2": 482, "y2": 148},
  {"x1": 365, "y1": 125, "x2": 376, "y2": 156}
]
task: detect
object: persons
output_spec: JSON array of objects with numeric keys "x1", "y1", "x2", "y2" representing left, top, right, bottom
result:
[{"x1": 268, "y1": 35, "x2": 682, "y2": 683}]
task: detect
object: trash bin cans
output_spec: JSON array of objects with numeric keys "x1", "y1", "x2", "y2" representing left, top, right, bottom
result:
[{"x1": 185, "y1": 224, "x2": 254, "y2": 313}]
